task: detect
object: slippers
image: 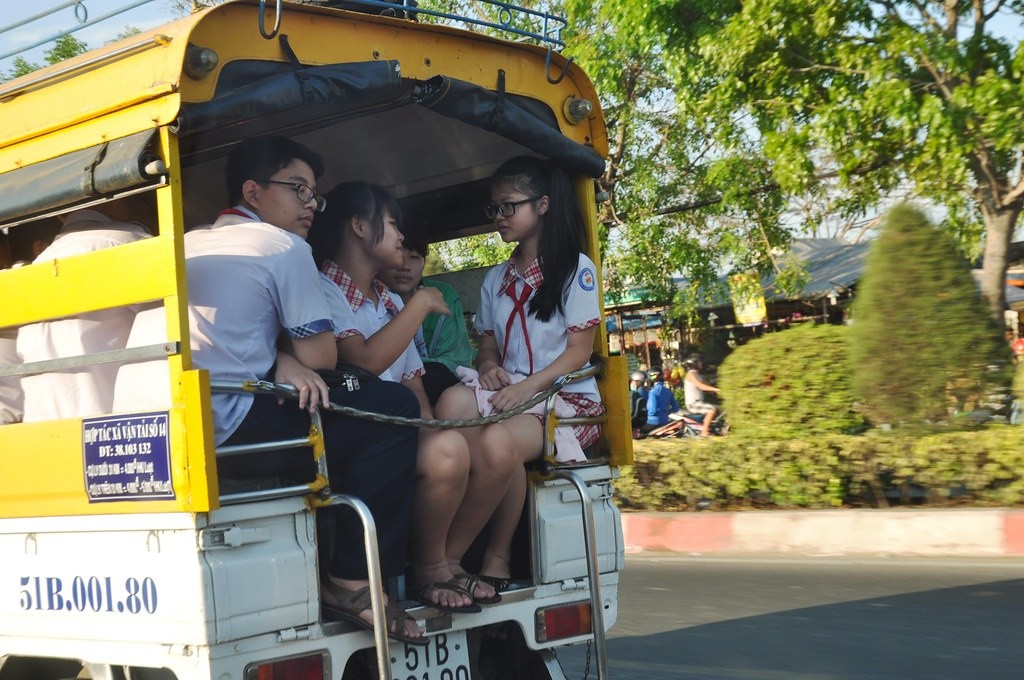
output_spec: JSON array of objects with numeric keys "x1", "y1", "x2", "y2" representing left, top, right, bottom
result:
[
  {"x1": 455, "y1": 571, "x2": 503, "y2": 604},
  {"x1": 413, "y1": 581, "x2": 483, "y2": 613}
]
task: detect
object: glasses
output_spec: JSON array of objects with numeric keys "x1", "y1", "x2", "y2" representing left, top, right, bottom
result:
[
  {"x1": 483, "y1": 196, "x2": 545, "y2": 220},
  {"x1": 252, "y1": 178, "x2": 327, "y2": 212}
]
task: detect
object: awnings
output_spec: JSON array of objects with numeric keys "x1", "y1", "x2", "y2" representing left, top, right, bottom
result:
[{"x1": 601, "y1": 242, "x2": 870, "y2": 314}]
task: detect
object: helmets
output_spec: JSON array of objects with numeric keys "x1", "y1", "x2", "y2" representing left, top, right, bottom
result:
[
  {"x1": 686, "y1": 353, "x2": 703, "y2": 369},
  {"x1": 631, "y1": 370, "x2": 647, "y2": 381},
  {"x1": 648, "y1": 367, "x2": 664, "y2": 382}
]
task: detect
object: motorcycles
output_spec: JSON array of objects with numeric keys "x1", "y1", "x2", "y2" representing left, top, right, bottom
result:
[{"x1": 632, "y1": 396, "x2": 731, "y2": 441}]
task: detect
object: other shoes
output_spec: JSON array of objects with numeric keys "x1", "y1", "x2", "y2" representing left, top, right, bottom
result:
[{"x1": 477, "y1": 572, "x2": 514, "y2": 591}]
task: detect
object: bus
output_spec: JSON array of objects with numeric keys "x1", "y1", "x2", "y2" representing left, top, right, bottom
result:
[{"x1": 0, "y1": 0, "x2": 635, "y2": 680}]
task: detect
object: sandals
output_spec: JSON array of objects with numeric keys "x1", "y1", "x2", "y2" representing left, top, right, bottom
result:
[{"x1": 320, "y1": 578, "x2": 430, "y2": 646}]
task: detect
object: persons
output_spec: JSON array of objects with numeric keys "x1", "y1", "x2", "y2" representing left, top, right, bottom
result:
[
  {"x1": 630, "y1": 354, "x2": 722, "y2": 441},
  {"x1": 0, "y1": 137, "x2": 599, "y2": 646},
  {"x1": 1003, "y1": 309, "x2": 1024, "y2": 356}
]
